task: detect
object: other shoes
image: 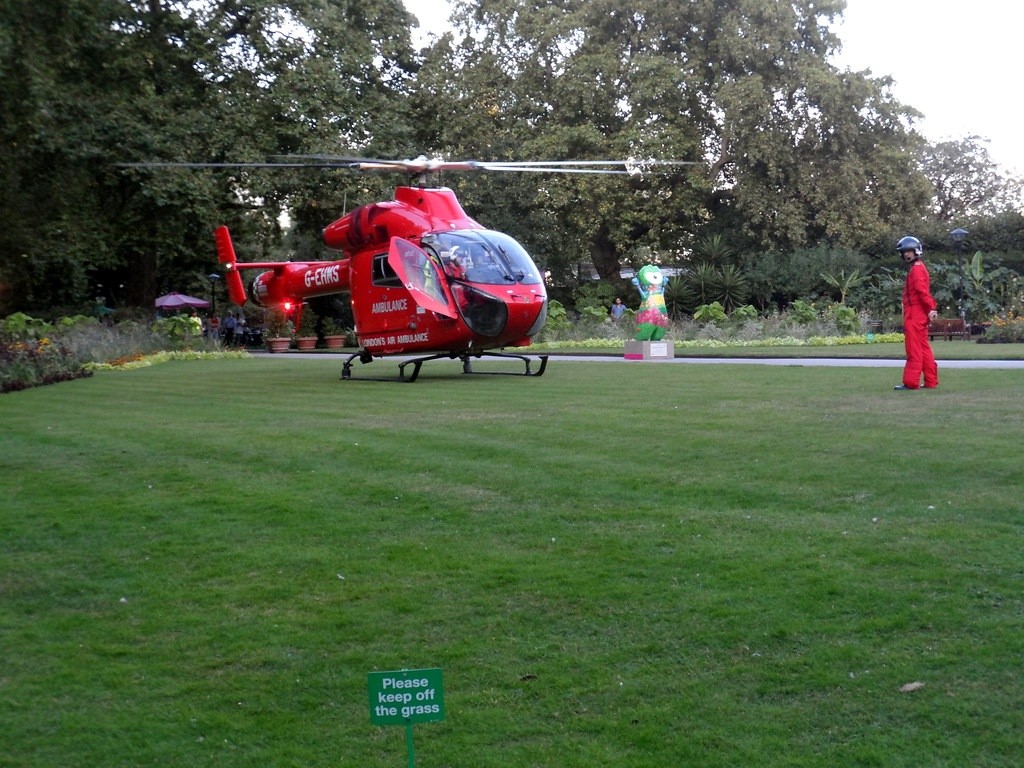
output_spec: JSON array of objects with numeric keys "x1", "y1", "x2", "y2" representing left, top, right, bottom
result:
[
  {"x1": 920, "y1": 384, "x2": 925, "y2": 388},
  {"x1": 895, "y1": 385, "x2": 910, "y2": 390}
]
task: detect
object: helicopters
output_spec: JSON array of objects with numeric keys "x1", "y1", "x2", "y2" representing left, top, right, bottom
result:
[{"x1": 114, "y1": 152, "x2": 705, "y2": 384}]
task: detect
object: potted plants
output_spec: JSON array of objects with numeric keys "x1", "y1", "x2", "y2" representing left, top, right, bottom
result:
[
  {"x1": 265, "y1": 308, "x2": 292, "y2": 353},
  {"x1": 321, "y1": 316, "x2": 347, "y2": 348},
  {"x1": 295, "y1": 304, "x2": 318, "y2": 350}
]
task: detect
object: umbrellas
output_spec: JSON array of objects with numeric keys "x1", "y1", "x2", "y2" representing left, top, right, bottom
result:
[{"x1": 155, "y1": 292, "x2": 210, "y2": 317}]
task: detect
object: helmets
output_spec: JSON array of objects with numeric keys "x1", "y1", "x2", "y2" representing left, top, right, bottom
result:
[
  {"x1": 896, "y1": 236, "x2": 922, "y2": 257},
  {"x1": 449, "y1": 246, "x2": 468, "y2": 262}
]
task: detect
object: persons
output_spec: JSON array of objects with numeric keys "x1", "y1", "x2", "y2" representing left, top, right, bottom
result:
[
  {"x1": 436, "y1": 245, "x2": 497, "y2": 329},
  {"x1": 188, "y1": 307, "x2": 247, "y2": 349},
  {"x1": 611, "y1": 298, "x2": 627, "y2": 321},
  {"x1": 892, "y1": 236, "x2": 939, "y2": 390}
]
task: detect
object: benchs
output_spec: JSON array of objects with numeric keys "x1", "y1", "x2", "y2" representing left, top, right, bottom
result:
[{"x1": 928, "y1": 319, "x2": 970, "y2": 341}]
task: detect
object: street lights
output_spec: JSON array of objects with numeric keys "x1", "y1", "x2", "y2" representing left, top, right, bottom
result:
[
  {"x1": 950, "y1": 229, "x2": 968, "y2": 316},
  {"x1": 208, "y1": 274, "x2": 218, "y2": 316}
]
